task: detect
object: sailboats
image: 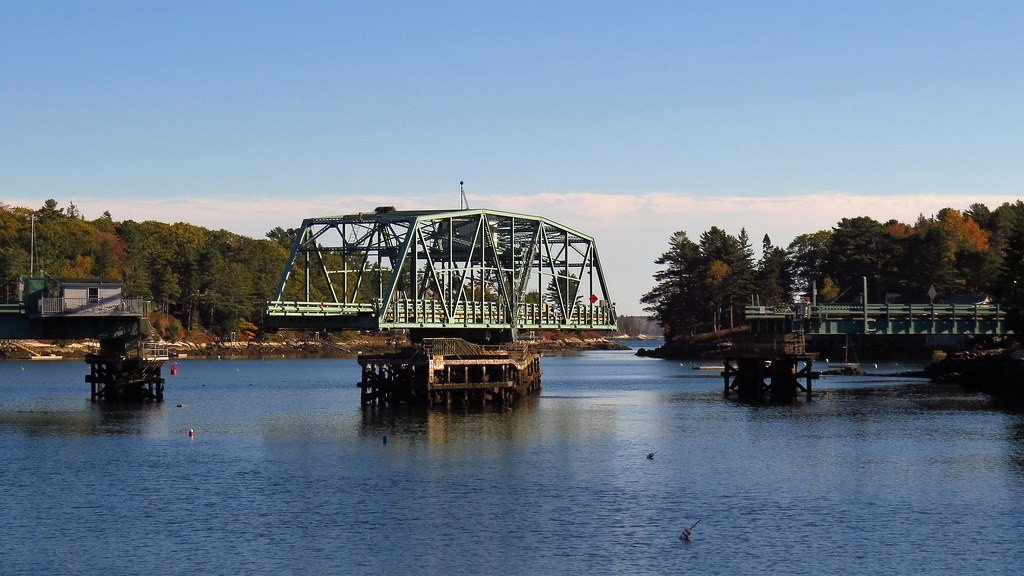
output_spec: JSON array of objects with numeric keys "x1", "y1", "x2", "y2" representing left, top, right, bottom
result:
[{"x1": 825, "y1": 334, "x2": 861, "y2": 369}]
